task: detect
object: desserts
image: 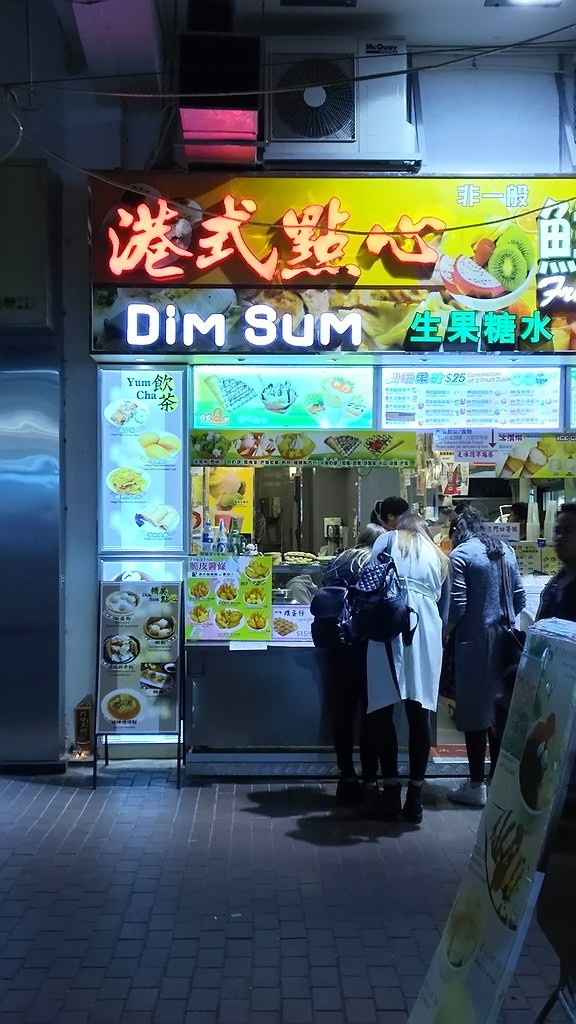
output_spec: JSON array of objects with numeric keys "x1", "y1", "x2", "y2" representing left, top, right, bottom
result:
[
  {"x1": 189, "y1": 431, "x2": 393, "y2": 461},
  {"x1": 205, "y1": 375, "x2": 298, "y2": 413}
]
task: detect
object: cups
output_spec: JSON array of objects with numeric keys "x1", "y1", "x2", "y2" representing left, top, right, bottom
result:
[
  {"x1": 544, "y1": 500, "x2": 558, "y2": 540},
  {"x1": 525, "y1": 502, "x2": 540, "y2": 542}
]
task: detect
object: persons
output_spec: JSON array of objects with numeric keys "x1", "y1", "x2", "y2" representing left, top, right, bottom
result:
[
  {"x1": 448, "y1": 504, "x2": 526, "y2": 810},
  {"x1": 533, "y1": 500, "x2": 576, "y2": 625},
  {"x1": 309, "y1": 496, "x2": 451, "y2": 824},
  {"x1": 506, "y1": 502, "x2": 528, "y2": 542}
]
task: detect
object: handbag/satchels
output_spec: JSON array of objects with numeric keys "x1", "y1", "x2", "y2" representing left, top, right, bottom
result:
[{"x1": 502, "y1": 627, "x2": 527, "y2": 660}]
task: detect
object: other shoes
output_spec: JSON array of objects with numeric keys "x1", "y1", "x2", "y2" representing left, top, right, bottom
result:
[
  {"x1": 337, "y1": 779, "x2": 357, "y2": 805},
  {"x1": 360, "y1": 781, "x2": 380, "y2": 804}
]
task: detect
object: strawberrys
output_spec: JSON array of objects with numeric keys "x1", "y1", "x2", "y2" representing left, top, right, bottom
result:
[{"x1": 331, "y1": 376, "x2": 354, "y2": 393}]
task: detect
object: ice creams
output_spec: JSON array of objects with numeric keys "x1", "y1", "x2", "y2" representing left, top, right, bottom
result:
[{"x1": 498, "y1": 446, "x2": 549, "y2": 479}]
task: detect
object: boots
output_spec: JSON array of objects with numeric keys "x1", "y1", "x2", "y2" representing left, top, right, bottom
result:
[
  {"x1": 402, "y1": 781, "x2": 423, "y2": 823},
  {"x1": 356, "y1": 782, "x2": 402, "y2": 821}
]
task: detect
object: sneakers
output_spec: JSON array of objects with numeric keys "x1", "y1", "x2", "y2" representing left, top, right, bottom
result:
[{"x1": 447, "y1": 778, "x2": 487, "y2": 805}]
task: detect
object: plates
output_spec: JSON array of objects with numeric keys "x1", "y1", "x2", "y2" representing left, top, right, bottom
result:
[
  {"x1": 188, "y1": 611, "x2": 210, "y2": 624},
  {"x1": 134, "y1": 504, "x2": 180, "y2": 534},
  {"x1": 215, "y1": 587, "x2": 239, "y2": 602},
  {"x1": 188, "y1": 586, "x2": 210, "y2": 599},
  {"x1": 246, "y1": 619, "x2": 269, "y2": 632},
  {"x1": 101, "y1": 634, "x2": 140, "y2": 665},
  {"x1": 242, "y1": 592, "x2": 265, "y2": 606},
  {"x1": 103, "y1": 398, "x2": 150, "y2": 427},
  {"x1": 105, "y1": 465, "x2": 152, "y2": 496},
  {"x1": 214, "y1": 608, "x2": 245, "y2": 632},
  {"x1": 316, "y1": 556, "x2": 336, "y2": 561},
  {"x1": 140, "y1": 671, "x2": 173, "y2": 689},
  {"x1": 243, "y1": 569, "x2": 270, "y2": 582},
  {"x1": 135, "y1": 429, "x2": 181, "y2": 459},
  {"x1": 144, "y1": 616, "x2": 176, "y2": 640},
  {"x1": 106, "y1": 590, "x2": 142, "y2": 616},
  {"x1": 101, "y1": 688, "x2": 147, "y2": 727}
]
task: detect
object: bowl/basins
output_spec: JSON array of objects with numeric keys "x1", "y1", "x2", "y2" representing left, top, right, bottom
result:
[
  {"x1": 516, "y1": 717, "x2": 562, "y2": 815},
  {"x1": 449, "y1": 261, "x2": 537, "y2": 311},
  {"x1": 444, "y1": 916, "x2": 482, "y2": 970},
  {"x1": 164, "y1": 663, "x2": 176, "y2": 673}
]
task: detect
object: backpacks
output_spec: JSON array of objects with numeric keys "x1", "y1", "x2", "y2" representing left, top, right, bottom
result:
[
  {"x1": 348, "y1": 530, "x2": 419, "y2": 646},
  {"x1": 310, "y1": 561, "x2": 352, "y2": 649}
]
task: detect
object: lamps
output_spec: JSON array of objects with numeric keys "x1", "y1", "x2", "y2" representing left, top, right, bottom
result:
[{"x1": 169, "y1": 108, "x2": 260, "y2": 166}]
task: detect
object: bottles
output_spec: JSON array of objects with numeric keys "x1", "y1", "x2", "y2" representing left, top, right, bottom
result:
[
  {"x1": 202, "y1": 522, "x2": 214, "y2": 553},
  {"x1": 216, "y1": 519, "x2": 228, "y2": 553},
  {"x1": 227, "y1": 516, "x2": 242, "y2": 553}
]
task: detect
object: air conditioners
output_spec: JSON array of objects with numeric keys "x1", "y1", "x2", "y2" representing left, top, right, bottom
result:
[{"x1": 258, "y1": 35, "x2": 417, "y2": 158}]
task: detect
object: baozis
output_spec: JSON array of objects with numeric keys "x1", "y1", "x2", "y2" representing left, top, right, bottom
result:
[{"x1": 111, "y1": 592, "x2": 136, "y2": 610}]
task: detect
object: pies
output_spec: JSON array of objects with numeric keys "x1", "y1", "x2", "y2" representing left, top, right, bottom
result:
[{"x1": 138, "y1": 505, "x2": 178, "y2": 532}]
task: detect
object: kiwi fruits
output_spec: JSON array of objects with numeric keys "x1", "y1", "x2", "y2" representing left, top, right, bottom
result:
[{"x1": 488, "y1": 228, "x2": 536, "y2": 292}]
